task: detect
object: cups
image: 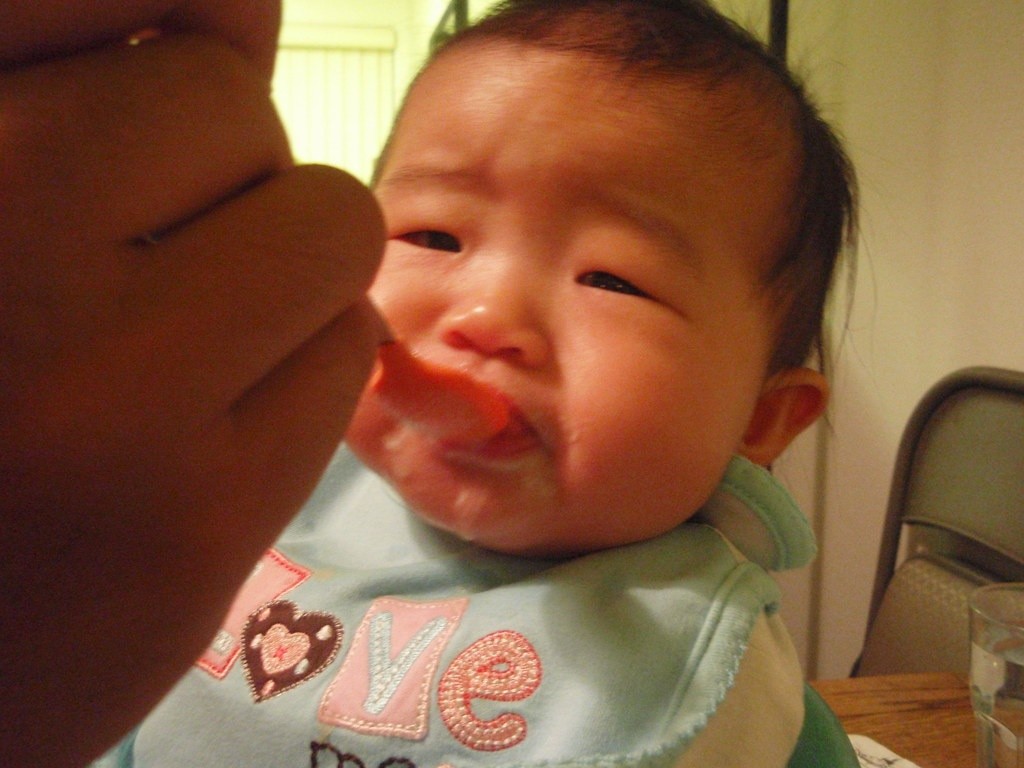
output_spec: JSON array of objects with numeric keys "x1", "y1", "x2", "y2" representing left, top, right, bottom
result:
[{"x1": 969, "y1": 582, "x2": 1024, "y2": 768}]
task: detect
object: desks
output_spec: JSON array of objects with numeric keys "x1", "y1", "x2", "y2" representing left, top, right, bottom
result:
[{"x1": 807, "y1": 671, "x2": 1024, "y2": 768}]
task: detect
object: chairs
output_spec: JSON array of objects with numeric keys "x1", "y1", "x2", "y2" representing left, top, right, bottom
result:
[{"x1": 848, "y1": 365, "x2": 1024, "y2": 679}]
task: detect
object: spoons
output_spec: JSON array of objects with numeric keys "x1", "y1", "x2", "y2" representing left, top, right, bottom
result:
[{"x1": 362, "y1": 294, "x2": 539, "y2": 455}]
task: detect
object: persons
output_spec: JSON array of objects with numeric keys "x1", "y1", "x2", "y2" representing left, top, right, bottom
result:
[
  {"x1": 0, "y1": 0, "x2": 388, "y2": 768},
  {"x1": 90, "y1": 0, "x2": 863, "y2": 768}
]
